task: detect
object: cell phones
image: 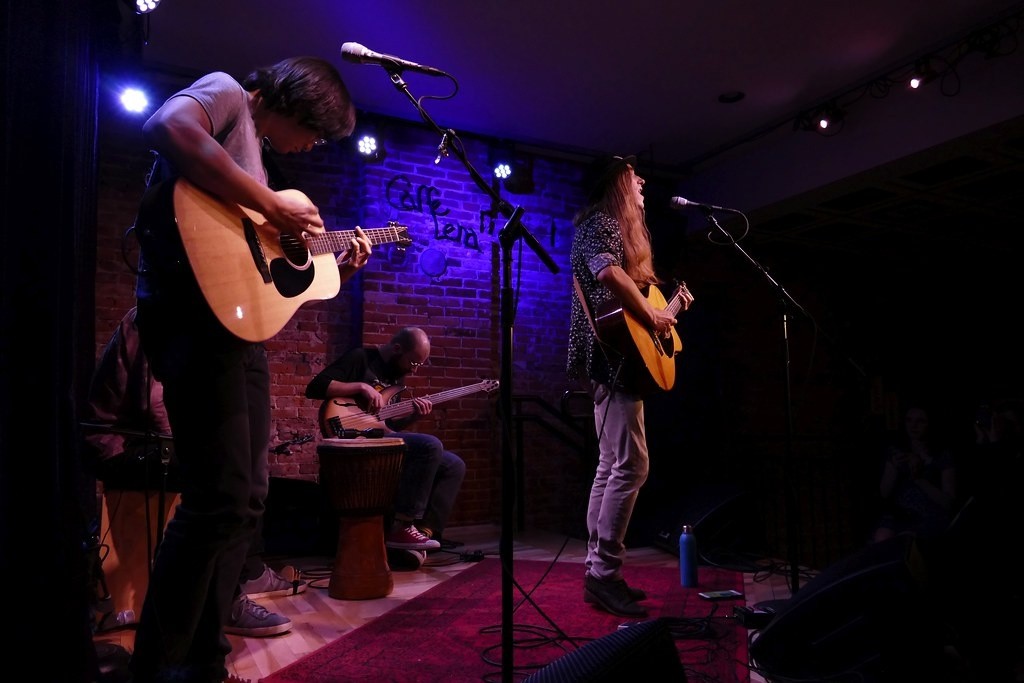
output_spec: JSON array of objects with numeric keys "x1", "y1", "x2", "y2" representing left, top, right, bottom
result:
[{"x1": 697, "y1": 590, "x2": 744, "y2": 600}]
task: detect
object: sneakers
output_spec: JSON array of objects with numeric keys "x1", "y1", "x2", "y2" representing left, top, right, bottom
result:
[
  {"x1": 239, "y1": 568, "x2": 307, "y2": 600},
  {"x1": 398, "y1": 550, "x2": 426, "y2": 571},
  {"x1": 222, "y1": 673, "x2": 266, "y2": 683},
  {"x1": 386, "y1": 524, "x2": 440, "y2": 548},
  {"x1": 223, "y1": 591, "x2": 292, "y2": 637}
]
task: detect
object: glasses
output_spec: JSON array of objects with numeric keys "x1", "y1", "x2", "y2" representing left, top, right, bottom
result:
[
  {"x1": 408, "y1": 359, "x2": 424, "y2": 366},
  {"x1": 311, "y1": 136, "x2": 327, "y2": 145}
]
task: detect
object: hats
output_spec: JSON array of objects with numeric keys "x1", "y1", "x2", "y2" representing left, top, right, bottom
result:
[{"x1": 579, "y1": 151, "x2": 636, "y2": 202}]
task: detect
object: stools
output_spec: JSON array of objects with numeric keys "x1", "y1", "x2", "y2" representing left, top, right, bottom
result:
[{"x1": 94, "y1": 469, "x2": 183, "y2": 629}]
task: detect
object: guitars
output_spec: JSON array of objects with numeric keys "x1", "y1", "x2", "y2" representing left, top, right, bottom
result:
[
  {"x1": 319, "y1": 375, "x2": 501, "y2": 443},
  {"x1": 593, "y1": 280, "x2": 697, "y2": 398},
  {"x1": 134, "y1": 170, "x2": 413, "y2": 355}
]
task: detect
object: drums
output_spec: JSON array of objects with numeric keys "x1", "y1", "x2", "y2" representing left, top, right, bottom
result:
[{"x1": 316, "y1": 436, "x2": 406, "y2": 600}]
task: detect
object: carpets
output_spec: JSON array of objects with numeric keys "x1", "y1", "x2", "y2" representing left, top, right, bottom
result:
[{"x1": 258, "y1": 557, "x2": 752, "y2": 683}]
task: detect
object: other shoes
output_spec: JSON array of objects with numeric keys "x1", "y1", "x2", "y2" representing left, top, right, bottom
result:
[{"x1": 583, "y1": 573, "x2": 648, "y2": 618}]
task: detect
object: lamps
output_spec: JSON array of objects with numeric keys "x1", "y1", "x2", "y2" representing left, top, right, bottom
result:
[{"x1": 496, "y1": 156, "x2": 535, "y2": 194}]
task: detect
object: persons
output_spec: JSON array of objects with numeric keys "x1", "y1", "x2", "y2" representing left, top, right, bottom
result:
[
  {"x1": 128, "y1": 56, "x2": 374, "y2": 683},
  {"x1": 565, "y1": 155, "x2": 695, "y2": 619},
  {"x1": 305, "y1": 327, "x2": 467, "y2": 570},
  {"x1": 873, "y1": 400, "x2": 1024, "y2": 544},
  {"x1": 77, "y1": 307, "x2": 309, "y2": 636}
]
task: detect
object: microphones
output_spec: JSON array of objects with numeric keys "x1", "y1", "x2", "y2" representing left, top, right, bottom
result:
[
  {"x1": 669, "y1": 197, "x2": 740, "y2": 213},
  {"x1": 341, "y1": 42, "x2": 446, "y2": 77},
  {"x1": 338, "y1": 428, "x2": 385, "y2": 439}
]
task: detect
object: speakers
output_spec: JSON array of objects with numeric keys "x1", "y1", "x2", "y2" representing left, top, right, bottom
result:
[
  {"x1": 746, "y1": 529, "x2": 950, "y2": 683},
  {"x1": 655, "y1": 488, "x2": 770, "y2": 568},
  {"x1": 516, "y1": 622, "x2": 692, "y2": 683},
  {"x1": 92, "y1": 479, "x2": 186, "y2": 634}
]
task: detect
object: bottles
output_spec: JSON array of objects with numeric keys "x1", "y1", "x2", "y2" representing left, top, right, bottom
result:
[{"x1": 679, "y1": 525, "x2": 698, "y2": 588}]
